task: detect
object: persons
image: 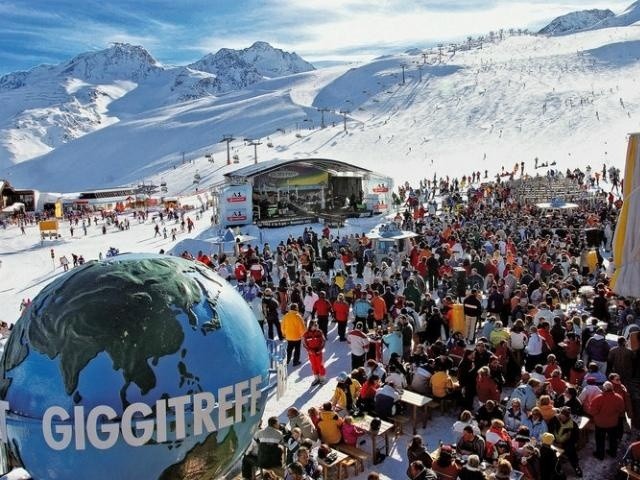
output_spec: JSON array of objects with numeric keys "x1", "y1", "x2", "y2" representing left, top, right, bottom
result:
[{"x1": 0, "y1": 156, "x2": 640, "y2": 479}]
